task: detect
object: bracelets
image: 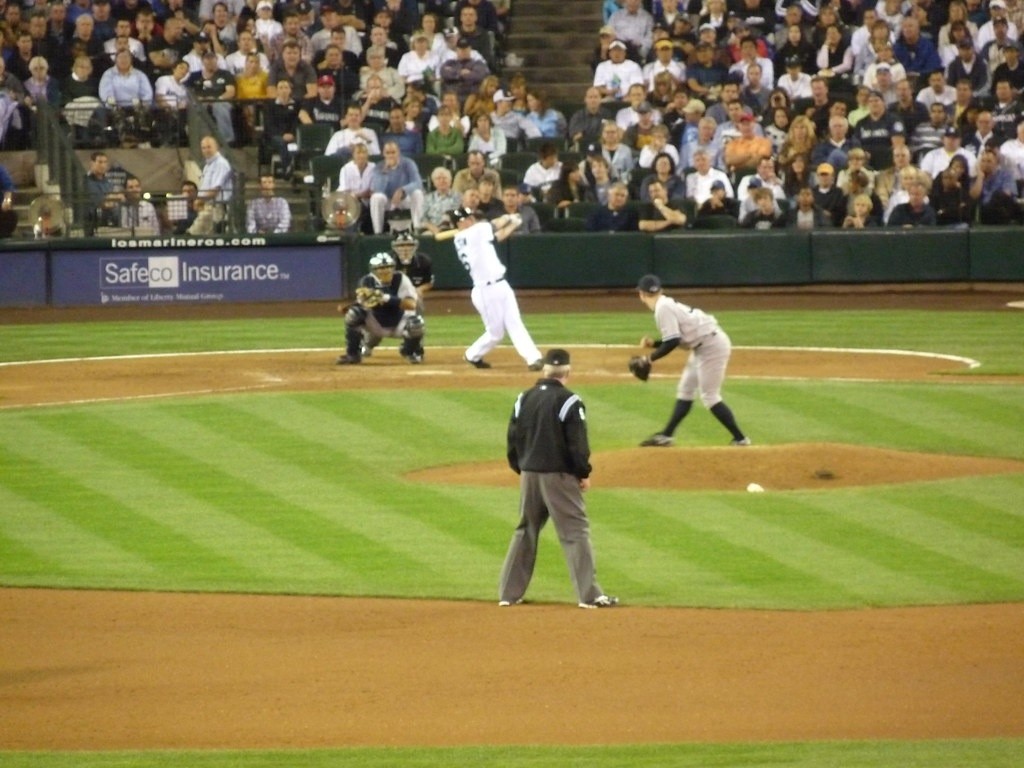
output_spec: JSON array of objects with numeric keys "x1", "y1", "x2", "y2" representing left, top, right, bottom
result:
[
  {"x1": 386, "y1": 295, "x2": 401, "y2": 308},
  {"x1": 3, "y1": 198, "x2": 11, "y2": 202},
  {"x1": 658, "y1": 206, "x2": 667, "y2": 212}
]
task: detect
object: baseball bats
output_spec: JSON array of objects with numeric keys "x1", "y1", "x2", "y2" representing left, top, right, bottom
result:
[{"x1": 435, "y1": 212, "x2": 521, "y2": 241}]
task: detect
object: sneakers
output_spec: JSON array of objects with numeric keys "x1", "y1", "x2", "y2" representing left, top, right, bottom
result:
[
  {"x1": 638, "y1": 433, "x2": 674, "y2": 446},
  {"x1": 461, "y1": 354, "x2": 491, "y2": 369},
  {"x1": 528, "y1": 358, "x2": 544, "y2": 372},
  {"x1": 578, "y1": 594, "x2": 619, "y2": 609},
  {"x1": 359, "y1": 347, "x2": 373, "y2": 357},
  {"x1": 498, "y1": 596, "x2": 527, "y2": 606},
  {"x1": 337, "y1": 351, "x2": 363, "y2": 365},
  {"x1": 400, "y1": 347, "x2": 424, "y2": 364},
  {"x1": 728, "y1": 437, "x2": 752, "y2": 446}
]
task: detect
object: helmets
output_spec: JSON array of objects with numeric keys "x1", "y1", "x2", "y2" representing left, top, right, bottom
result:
[
  {"x1": 515, "y1": 182, "x2": 533, "y2": 195},
  {"x1": 633, "y1": 100, "x2": 653, "y2": 112},
  {"x1": 391, "y1": 232, "x2": 420, "y2": 252},
  {"x1": 368, "y1": 252, "x2": 397, "y2": 270},
  {"x1": 450, "y1": 206, "x2": 475, "y2": 225}
]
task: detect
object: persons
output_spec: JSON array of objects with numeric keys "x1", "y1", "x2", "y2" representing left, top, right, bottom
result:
[
  {"x1": 336, "y1": 232, "x2": 434, "y2": 367},
  {"x1": 0, "y1": 0, "x2": 1024, "y2": 240},
  {"x1": 451, "y1": 207, "x2": 550, "y2": 371},
  {"x1": 629, "y1": 274, "x2": 750, "y2": 447},
  {"x1": 499, "y1": 349, "x2": 619, "y2": 608}
]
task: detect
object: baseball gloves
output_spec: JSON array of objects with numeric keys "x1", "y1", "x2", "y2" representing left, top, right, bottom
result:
[
  {"x1": 628, "y1": 355, "x2": 652, "y2": 381},
  {"x1": 355, "y1": 286, "x2": 384, "y2": 308}
]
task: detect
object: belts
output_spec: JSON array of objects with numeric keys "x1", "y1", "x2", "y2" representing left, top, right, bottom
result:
[{"x1": 487, "y1": 277, "x2": 506, "y2": 286}]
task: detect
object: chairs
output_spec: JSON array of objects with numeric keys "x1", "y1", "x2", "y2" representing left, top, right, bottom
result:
[{"x1": 290, "y1": 120, "x2": 738, "y2": 230}]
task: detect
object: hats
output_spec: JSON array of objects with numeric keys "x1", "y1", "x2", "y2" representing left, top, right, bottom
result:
[
  {"x1": 543, "y1": 349, "x2": 571, "y2": 365},
  {"x1": 617, "y1": 0, "x2": 1023, "y2": 193},
  {"x1": 607, "y1": 39, "x2": 628, "y2": 51},
  {"x1": 193, "y1": 2, "x2": 557, "y2": 104},
  {"x1": 634, "y1": 274, "x2": 662, "y2": 294}
]
